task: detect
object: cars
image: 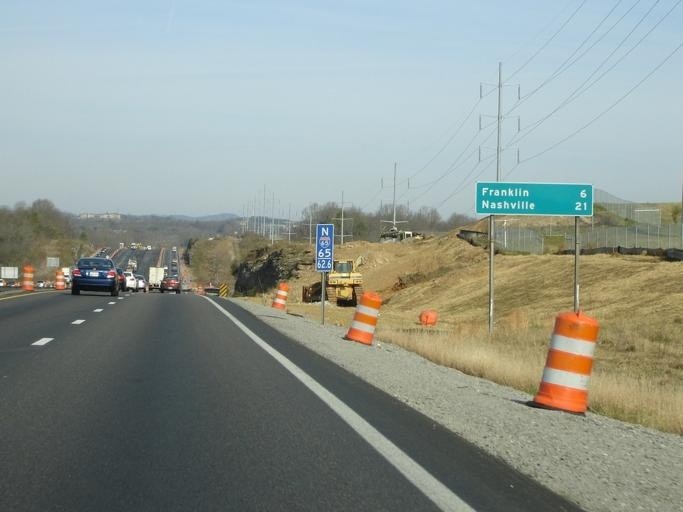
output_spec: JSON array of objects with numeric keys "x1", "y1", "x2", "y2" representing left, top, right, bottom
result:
[{"x1": 0, "y1": 242, "x2": 191, "y2": 296}]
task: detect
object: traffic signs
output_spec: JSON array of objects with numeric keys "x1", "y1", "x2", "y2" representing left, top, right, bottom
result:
[{"x1": 316, "y1": 224, "x2": 333, "y2": 270}]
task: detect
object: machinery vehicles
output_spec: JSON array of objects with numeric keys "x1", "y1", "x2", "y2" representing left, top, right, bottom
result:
[
  {"x1": 381, "y1": 228, "x2": 422, "y2": 245},
  {"x1": 302, "y1": 257, "x2": 363, "y2": 308}
]
